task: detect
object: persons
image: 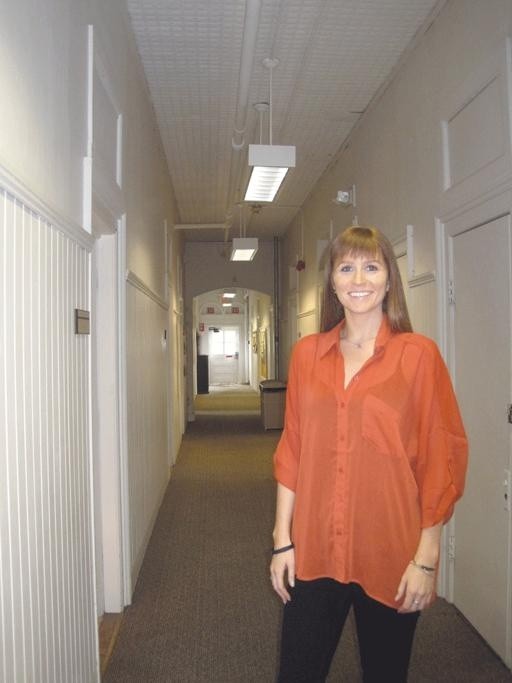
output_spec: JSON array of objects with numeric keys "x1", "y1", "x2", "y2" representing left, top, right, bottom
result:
[{"x1": 269, "y1": 227, "x2": 468, "y2": 683}]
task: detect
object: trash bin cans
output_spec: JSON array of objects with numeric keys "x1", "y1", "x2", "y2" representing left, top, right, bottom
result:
[{"x1": 259, "y1": 380, "x2": 287, "y2": 431}]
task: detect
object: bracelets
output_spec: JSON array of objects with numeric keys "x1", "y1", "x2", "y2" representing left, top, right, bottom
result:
[
  {"x1": 271, "y1": 542, "x2": 295, "y2": 555},
  {"x1": 410, "y1": 559, "x2": 437, "y2": 578}
]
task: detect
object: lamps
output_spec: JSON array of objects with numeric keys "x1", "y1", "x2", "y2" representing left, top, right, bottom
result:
[
  {"x1": 222, "y1": 287, "x2": 238, "y2": 298},
  {"x1": 235, "y1": 56, "x2": 296, "y2": 205},
  {"x1": 228, "y1": 183, "x2": 259, "y2": 261},
  {"x1": 221, "y1": 300, "x2": 233, "y2": 307}
]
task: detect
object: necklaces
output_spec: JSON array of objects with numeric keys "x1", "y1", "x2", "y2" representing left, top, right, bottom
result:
[{"x1": 346, "y1": 338, "x2": 372, "y2": 350}]
